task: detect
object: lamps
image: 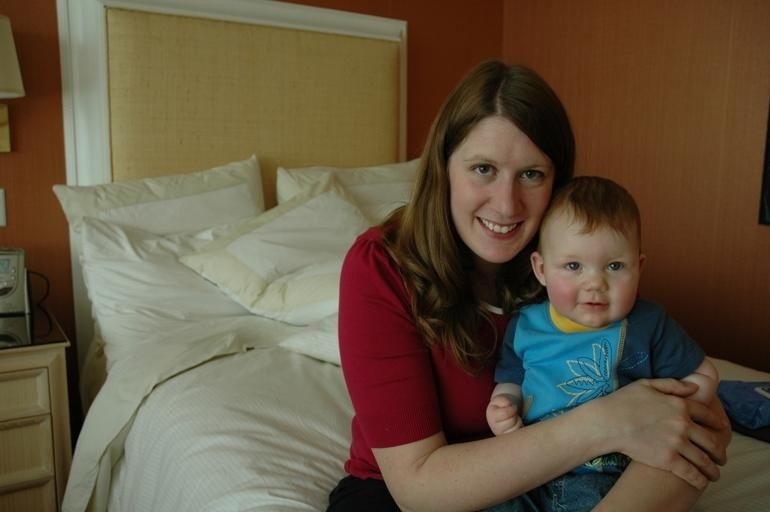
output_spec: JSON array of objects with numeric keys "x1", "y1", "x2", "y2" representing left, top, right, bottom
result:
[{"x1": 0, "y1": 15, "x2": 25, "y2": 153}]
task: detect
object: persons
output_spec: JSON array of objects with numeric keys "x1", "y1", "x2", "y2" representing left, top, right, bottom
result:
[
  {"x1": 483, "y1": 173, "x2": 721, "y2": 512},
  {"x1": 324, "y1": 57, "x2": 734, "y2": 511}
]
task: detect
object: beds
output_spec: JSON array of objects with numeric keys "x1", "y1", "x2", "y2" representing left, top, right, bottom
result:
[{"x1": 55, "y1": 1, "x2": 770, "y2": 512}]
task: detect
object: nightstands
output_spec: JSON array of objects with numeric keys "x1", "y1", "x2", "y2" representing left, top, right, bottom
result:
[{"x1": 0, "y1": 309, "x2": 75, "y2": 512}]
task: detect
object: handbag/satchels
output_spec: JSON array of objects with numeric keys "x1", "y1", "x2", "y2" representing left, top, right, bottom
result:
[{"x1": 717, "y1": 380, "x2": 770, "y2": 443}]
